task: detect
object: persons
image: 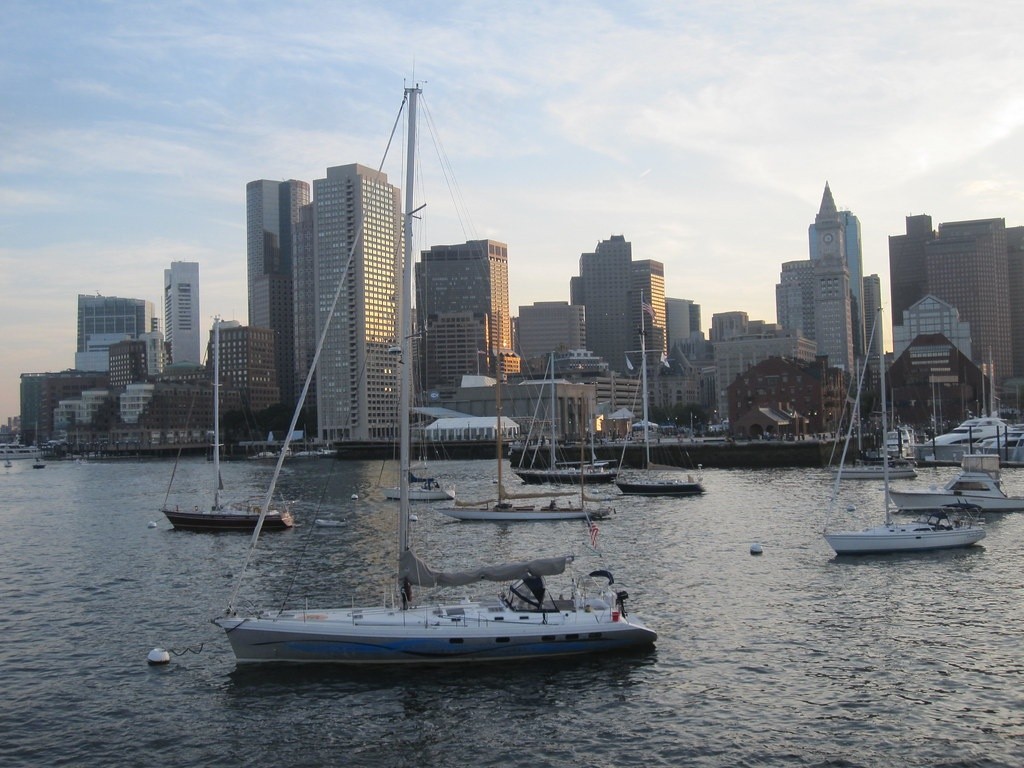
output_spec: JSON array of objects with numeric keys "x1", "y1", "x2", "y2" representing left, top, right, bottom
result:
[
  {"x1": 550, "y1": 500, "x2": 557, "y2": 512},
  {"x1": 569, "y1": 501, "x2": 575, "y2": 512},
  {"x1": 524, "y1": 435, "x2": 546, "y2": 445},
  {"x1": 759, "y1": 430, "x2": 794, "y2": 441}
]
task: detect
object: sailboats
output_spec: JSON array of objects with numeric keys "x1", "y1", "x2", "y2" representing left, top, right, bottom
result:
[
  {"x1": 209, "y1": 84, "x2": 657, "y2": 665},
  {"x1": 377, "y1": 341, "x2": 456, "y2": 501},
  {"x1": 822, "y1": 308, "x2": 986, "y2": 554},
  {"x1": 513, "y1": 351, "x2": 618, "y2": 484},
  {"x1": 160, "y1": 318, "x2": 294, "y2": 530},
  {"x1": 32, "y1": 421, "x2": 45, "y2": 469},
  {"x1": 616, "y1": 354, "x2": 707, "y2": 496},
  {"x1": 828, "y1": 359, "x2": 918, "y2": 480},
  {"x1": 434, "y1": 309, "x2": 612, "y2": 520}
]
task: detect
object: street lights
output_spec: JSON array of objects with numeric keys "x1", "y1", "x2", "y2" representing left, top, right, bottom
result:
[{"x1": 808, "y1": 412, "x2": 817, "y2": 437}]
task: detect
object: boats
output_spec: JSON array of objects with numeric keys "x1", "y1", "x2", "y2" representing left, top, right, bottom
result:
[
  {"x1": 0, "y1": 444, "x2": 41, "y2": 459},
  {"x1": 962, "y1": 430, "x2": 1024, "y2": 460},
  {"x1": 880, "y1": 426, "x2": 916, "y2": 458},
  {"x1": 248, "y1": 447, "x2": 328, "y2": 458},
  {"x1": 890, "y1": 454, "x2": 1024, "y2": 514},
  {"x1": 924, "y1": 418, "x2": 1013, "y2": 444}
]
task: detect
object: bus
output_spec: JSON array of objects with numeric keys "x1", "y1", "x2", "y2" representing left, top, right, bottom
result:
[{"x1": 633, "y1": 421, "x2": 658, "y2": 437}]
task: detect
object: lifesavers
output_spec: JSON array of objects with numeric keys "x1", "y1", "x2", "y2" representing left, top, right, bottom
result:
[{"x1": 254, "y1": 508, "x2": 259, "y2": 513}]
